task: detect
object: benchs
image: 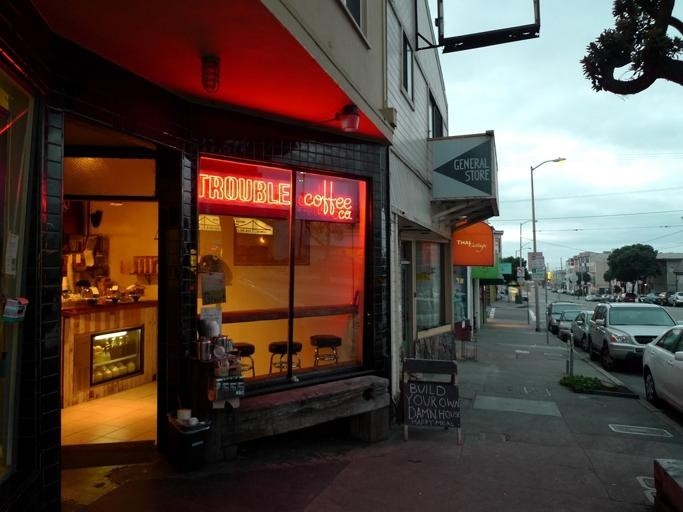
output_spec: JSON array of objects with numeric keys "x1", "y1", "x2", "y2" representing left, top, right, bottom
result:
[{"x1": 214, "y1": 372, "x2": 392, "y2": 476}]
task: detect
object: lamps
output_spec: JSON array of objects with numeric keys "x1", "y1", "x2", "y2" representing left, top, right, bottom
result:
[
  {"x1": 315, "y1": 102, "x2": 361, "y2": 134},
  {"x1": 199, "y1": 48, "x2": 222, "y2": 92}
]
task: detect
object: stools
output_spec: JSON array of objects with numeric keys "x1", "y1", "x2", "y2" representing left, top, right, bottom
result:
[{"x1": 231, "y1": 331, "x2": 342, "y2": 381}]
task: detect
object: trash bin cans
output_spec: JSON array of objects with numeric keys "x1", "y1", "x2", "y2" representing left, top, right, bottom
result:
[
  {"x1": 515, "y1": 295, "x2": 522, "y2": 304},
  {"x1": 164, "y1": 412, "x2": 214, "y2": 474}
]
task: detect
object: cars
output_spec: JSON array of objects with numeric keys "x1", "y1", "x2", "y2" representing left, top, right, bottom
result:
[
  {"x1": 642, "y1": 325, "x2": 683, "y2": 415},
  {"x1": 546, "y1": 300, "x2": 595, "y2": 355},
  {"x1": 585, "y1": 290, "x2": 683, "y2": 307},
  {"x1": 587, "y1": 301, "x2": 681, "y2": 373},
  {"x1": 499, "y1": 291, "x2": 529, "y2": 301}
]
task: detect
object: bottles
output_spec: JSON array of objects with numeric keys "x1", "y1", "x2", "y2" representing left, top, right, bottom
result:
[
  {"x1": 196, "y1": 321, "x2": 232, "y2": 361},
  {"x1": 90, "y1": 335, "x2": 140, "y2": 386}
]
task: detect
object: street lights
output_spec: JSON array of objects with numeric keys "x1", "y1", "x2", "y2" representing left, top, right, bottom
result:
[{"x1": 515, "y1": 157, "x2": 567, "y2": 332}]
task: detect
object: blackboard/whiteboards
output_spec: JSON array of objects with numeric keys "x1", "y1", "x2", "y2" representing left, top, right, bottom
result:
[{"x1": 404, "y1": 358, "x2": 461, "y2": 427}]
